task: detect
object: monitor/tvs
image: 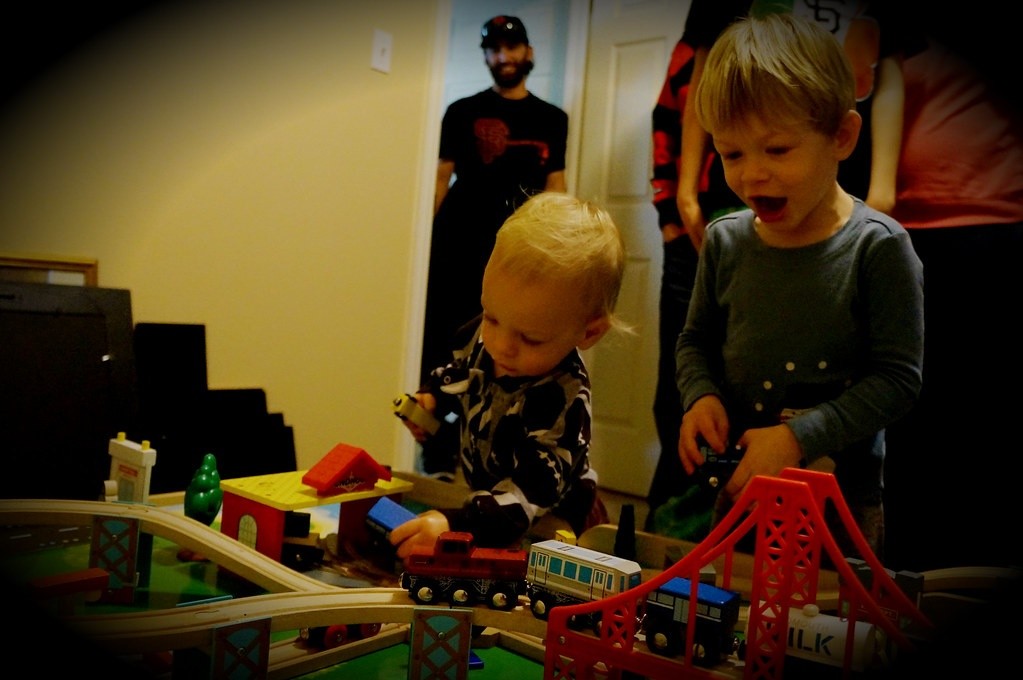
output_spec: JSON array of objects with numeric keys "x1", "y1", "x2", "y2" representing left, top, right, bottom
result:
[{"x1": 0, "y1": 279, "x2": 138, "y2": 478}]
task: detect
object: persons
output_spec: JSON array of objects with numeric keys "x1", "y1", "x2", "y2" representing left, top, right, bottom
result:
[
  {"x1": 644, "y1": 0, "x2": 1023, "y2": 574},
  {"x1": 676, "y1": 19, "x2": 925, "y2": 572},
  {"x1": 420, "y1": 16, "x2": 570, "y2": 475},
  {"x1": 390, "y1": 191, "x2": 626, "y2": 563}
]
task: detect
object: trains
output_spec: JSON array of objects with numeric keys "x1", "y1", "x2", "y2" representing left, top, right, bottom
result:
[{"x1": 401, "y1": 531, "x2": 885, "y2": 680}]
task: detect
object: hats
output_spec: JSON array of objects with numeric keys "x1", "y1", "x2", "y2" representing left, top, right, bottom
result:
[{"x1": 481, "y1": 15, "x2": 526, "y2": 48}]
